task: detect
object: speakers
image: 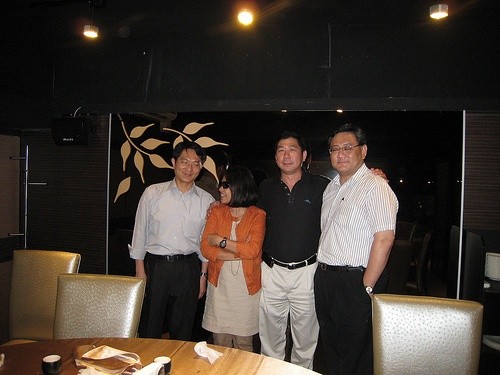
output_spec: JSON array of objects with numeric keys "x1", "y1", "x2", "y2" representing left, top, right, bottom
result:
[{"x1": 51, "y1": 115, "x2": 95, "y2": 145}]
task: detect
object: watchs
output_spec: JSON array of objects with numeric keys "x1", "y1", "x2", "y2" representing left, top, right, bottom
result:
[
  {"x1": 365, "y1": 285, "x2": 373, "y2": 293},
  {"x1": 219, "y1": 237, "x2": 227, "y2": 248}
]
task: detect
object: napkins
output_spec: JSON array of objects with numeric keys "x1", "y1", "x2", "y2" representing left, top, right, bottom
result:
[{"x1": 75, "y1": 346, "x2": 141, "y2": 374}]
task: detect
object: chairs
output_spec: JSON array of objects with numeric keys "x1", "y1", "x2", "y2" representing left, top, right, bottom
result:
[
  {"x1": 0, "y1": 247, "x2": 147, "y2": 338},
  {"x1": 370, "y1": 292, "x2": 484, "y2": 375},
  {"x1": 377, "y1": 222, "x2": 461, "y2": 296}
]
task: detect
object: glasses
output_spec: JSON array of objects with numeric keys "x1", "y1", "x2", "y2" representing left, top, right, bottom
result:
[
  {"x1": 219, "y1": 181, "x2": 230, "y2": 189},
  {"x1": 329, "y1": 144, "x2": 362, "y2": 155}
]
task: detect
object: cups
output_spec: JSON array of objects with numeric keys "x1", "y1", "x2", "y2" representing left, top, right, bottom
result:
[
  {"x1": 42, "y1": 354, "x2": 62, "y2": 375},
  {"x1": 153, "y1": 356, "x2": 171, "y2": 375}
]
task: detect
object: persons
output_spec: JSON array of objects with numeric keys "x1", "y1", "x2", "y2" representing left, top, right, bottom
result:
[
  {"x1": 200, "y1": 165, "x2": 266, "y2": 353},
  {"x1": 128, "y1": 142, "x2": 216, "y2": 341},
  {"x1": 206, "y1": 131, "x2": 389, "y2": 370},
  {"x1": 313, "y1": 123, "x2": 399, "y2": 375}
]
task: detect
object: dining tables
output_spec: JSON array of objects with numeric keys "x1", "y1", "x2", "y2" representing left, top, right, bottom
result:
[{"x1": 0, "y1": 338, "x2": 322, "y2": 374}]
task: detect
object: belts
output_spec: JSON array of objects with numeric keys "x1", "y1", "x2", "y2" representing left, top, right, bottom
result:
[
  {"x1": 316, "y1": 261, "x2": 364, "y2": 272},
  {"x1": 159, "y1": 253, "x2": 197, "y2": 262},
  {"x1": 270, "y1": 254, "x2": 317, "y2": 269}
]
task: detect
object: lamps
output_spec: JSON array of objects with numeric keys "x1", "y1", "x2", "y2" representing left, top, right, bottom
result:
[
  {"x1": 430, "y1": 4, "x2": 448, "y2": 20},
  {"x1": 83, "y1": 6, "x2": 99, "y2": 36}
]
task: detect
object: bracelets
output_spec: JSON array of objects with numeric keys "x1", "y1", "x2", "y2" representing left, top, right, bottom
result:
[{"x1": 200, "y1": 272, "x2": 208, "y2": 277}]
